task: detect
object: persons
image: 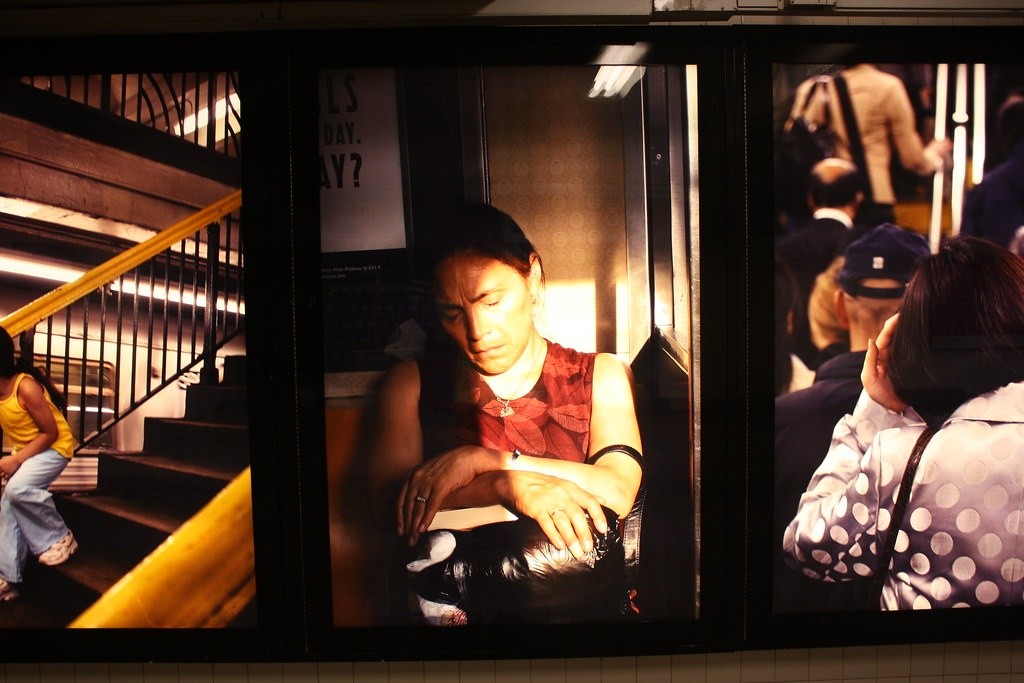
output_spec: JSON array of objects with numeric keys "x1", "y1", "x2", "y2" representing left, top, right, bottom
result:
[
  {"x1": 368, "y1": 206, "x2": 644, "y2": 622},
  {"x1": 774, "y1": 61, "x2": 1024, "y2": 612},
  {"x1": 0, "y1": 326, "x2": 78, "y2": 600}
]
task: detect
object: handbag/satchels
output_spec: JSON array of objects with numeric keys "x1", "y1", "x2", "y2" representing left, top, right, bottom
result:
[
  {"x1": 395, "y1": 444, "x2": 648, "y2": 659},
  {"x1": 772, "y1": 554, "x2": 883, "y2": 619},
  {"x1": 778, "y1": 77, "x2": 834, "y2": 183}
]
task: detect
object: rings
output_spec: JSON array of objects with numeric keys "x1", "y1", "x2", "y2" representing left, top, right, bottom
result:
[
  {"x1": 414, "y1": 495, "x2": 426, "y2": 503},
  {"x1": 550, "y1": 510, "x2": 566, "y2": 518}
]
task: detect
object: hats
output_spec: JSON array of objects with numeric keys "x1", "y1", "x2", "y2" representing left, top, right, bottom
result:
[{"x1": 839, "y1": 223, "x2": 931, "y2": 299}]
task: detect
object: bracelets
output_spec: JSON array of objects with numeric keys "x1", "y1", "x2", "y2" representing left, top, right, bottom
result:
[{"x1": 511, "y1": 449, "x2": 521, "y2": 471}]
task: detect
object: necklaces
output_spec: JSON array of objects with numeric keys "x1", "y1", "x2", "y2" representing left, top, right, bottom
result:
[{"x1": 478, "y1": 337, "x2": 543, "y2": 417}]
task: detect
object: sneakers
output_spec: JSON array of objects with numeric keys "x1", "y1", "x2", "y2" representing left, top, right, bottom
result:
[
  {"x1": 38, "y1": 529, "x2": 78, "y2": 566},
  {"x1": 0, "y1": 576, "x2": 18, "y2": 599}
]
